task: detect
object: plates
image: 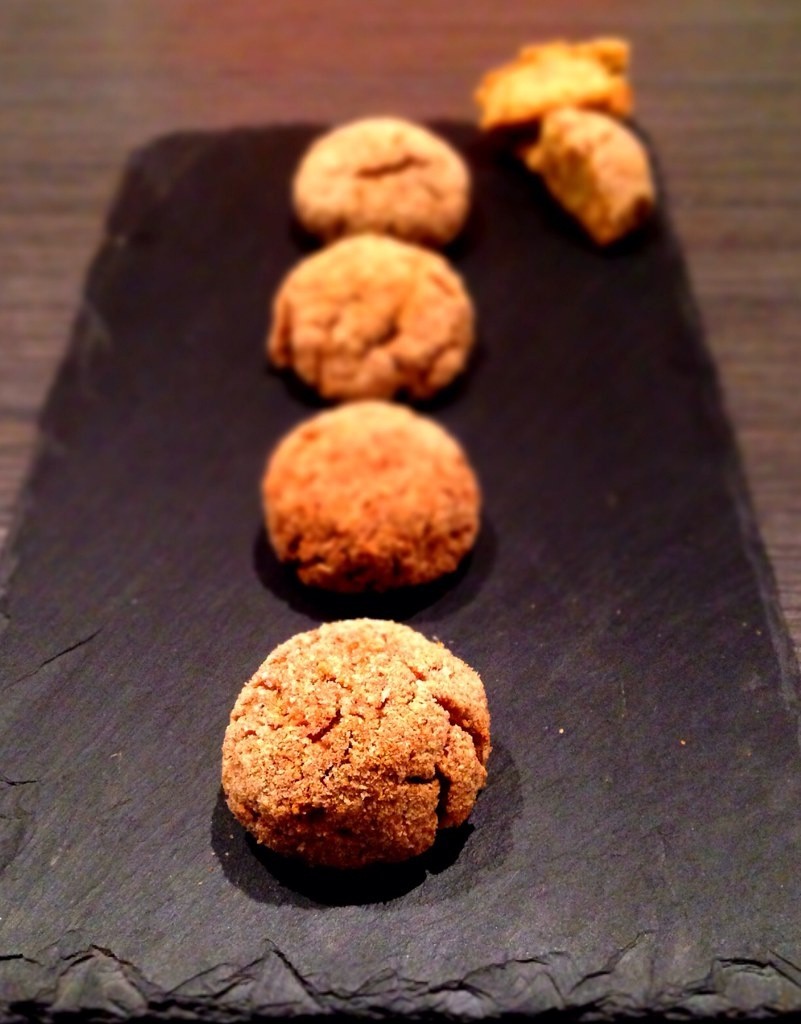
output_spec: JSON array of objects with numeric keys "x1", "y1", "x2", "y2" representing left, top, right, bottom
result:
[{"x1": 0, "y1": 108, "x2": 801, "y2": 1024}]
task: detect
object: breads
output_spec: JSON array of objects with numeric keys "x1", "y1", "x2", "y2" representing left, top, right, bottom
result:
[
  {"x1": 222, "y1": 615, "x2": 490, "y2": 867},
  {"x1": 291, "y1": 115, "x2": 469, "y2": 243},
  {"x1": 526, "y1": 106, "x2": 653, "y2": 240},
  {"x1": 269, "y1": 233, "x2": 471, "y2": 400},
  {"x1": 258, "y1": 401, "x2": 480, "y2": 593}
]
